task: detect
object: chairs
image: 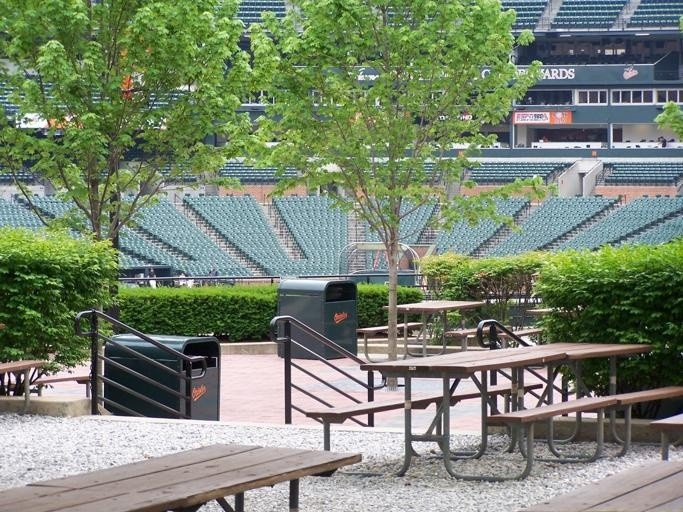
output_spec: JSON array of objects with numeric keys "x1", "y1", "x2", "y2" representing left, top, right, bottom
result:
[{"x1": 0, "y1": 1, "x2": 683, "y2": 278}]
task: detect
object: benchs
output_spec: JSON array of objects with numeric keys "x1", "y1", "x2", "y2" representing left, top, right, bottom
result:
[
  {"x1": 555, "y1": 384, "x2": 683, "y2": 459},
  {"x1": 4, "y1": 374, "x2": 91, "y2": 397},
  {"x1": 0, "y1": 360, "x2": 46, "y2": 414},
  {"x1": 448, "y1": 397, "x2": 618, "y2": 482},
  {"x1": 356, "y1": 322, "x2": 421, "y2": 361},
  {"x1": 649, "y1": 412, "x2": 683, "y2": 459},
  {"x1": 446, "y1": 325, "x2": 511, "y2": 351},
  {"x1": 440, "y1": 384, "x2": 543, "y2": 454},
  {"x1": 305, "y1": 392, "x2": 450, "y2": 450},
  {"x1": 497, "y1": 328, "x2": 544, "y2": 348}
]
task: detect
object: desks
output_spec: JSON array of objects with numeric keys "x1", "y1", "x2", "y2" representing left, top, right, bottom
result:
[
  {"x1": 0, "y1": 444, "x2": 361, "y2": 511},
  {"x1": 487, "y1": 342, "x2": 652, "y2": 457},
  {"x1": 360, "y1": 351, "x2": 567, "y2": 476},
  {"x1": 526, "y1": 308, "x2": 578, "y2": 342},
  {"x1": 510, "y1": 460, "x2": 682, "y2": 512},
  {"x1": 383, "y1": 300, "x2": 484, "y2": 358}
]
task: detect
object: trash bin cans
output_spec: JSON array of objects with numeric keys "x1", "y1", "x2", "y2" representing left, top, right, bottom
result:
[
  {"x1": 104, "y1": 334, "x2": 221, "y2": 422},
  {"x1": 278, "y1": 278, "x2": 357, "y2": 359}
]
